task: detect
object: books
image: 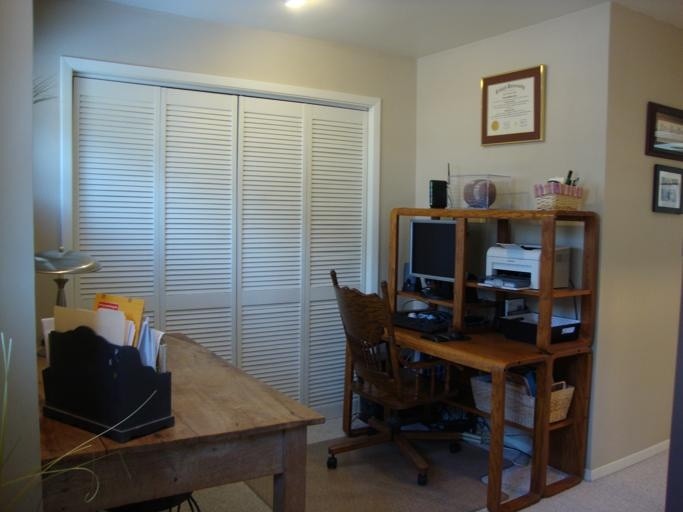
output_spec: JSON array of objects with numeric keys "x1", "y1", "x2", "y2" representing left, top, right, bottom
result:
[{"x1": 52, "y1": 294, "x2": 168, "y2": 377}]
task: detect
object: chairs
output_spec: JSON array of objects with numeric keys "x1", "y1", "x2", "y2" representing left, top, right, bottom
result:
[{"x1": 326, "y1": 269, "x2": 466, "y2": 484}]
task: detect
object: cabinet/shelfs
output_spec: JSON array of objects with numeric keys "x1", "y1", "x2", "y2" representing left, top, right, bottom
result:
[{"x1": 340, "y1": 206, "x2": 599, "y2": 512}]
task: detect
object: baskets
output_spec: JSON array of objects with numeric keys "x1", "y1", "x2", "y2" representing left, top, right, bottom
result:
[
  {"x1": 470, "y1": 370, "x2": 576, "y2": 429},
  {"x1": 533, "y1": 182, "x2": 585, "y2": 212}
]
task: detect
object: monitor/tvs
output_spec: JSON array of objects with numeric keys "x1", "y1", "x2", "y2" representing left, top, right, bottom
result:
[{"x1": 409, "y1": 220, "x2": 456, "y2": 299}]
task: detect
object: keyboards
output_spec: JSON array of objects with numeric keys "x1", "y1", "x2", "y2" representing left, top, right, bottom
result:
[{"x1": 391, "y1": 318, "x2": 448, "y2": 334}]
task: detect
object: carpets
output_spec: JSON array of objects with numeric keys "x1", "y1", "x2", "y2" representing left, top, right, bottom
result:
[{"x1": 244, "y1": 424, "x2": 515, "y2": 512}]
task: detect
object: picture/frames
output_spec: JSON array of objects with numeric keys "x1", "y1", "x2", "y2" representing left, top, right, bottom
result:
[
  {"x1": 478, "y1": 65, "x2": 547, "y2": 146},
  {"x1": 645, "y1": 99, "x2": 683, "y2": 214}
]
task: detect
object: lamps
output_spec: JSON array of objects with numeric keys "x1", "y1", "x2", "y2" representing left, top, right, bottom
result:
[{"x1": 35, "y1": 246, "x2": 102, "y2": 309}]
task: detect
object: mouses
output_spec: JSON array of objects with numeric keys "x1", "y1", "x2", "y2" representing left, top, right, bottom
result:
[{"x1": 451, "y1": 329, "x2": 463, "y2": 340}]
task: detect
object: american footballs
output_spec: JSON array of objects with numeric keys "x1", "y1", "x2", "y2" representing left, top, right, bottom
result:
[{"x1": 464, "y1": 179, "x2": 496, "y2": 208}]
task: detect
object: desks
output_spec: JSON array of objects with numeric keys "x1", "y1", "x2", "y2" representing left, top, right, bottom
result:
[{"x1": 34, "y1": 331, "x2": 327, "y2": 512}]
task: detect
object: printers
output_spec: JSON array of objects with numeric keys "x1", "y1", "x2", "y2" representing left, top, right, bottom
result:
[{"x1": 477, "y1": 243, "x2": 570, "y2": 291}]
